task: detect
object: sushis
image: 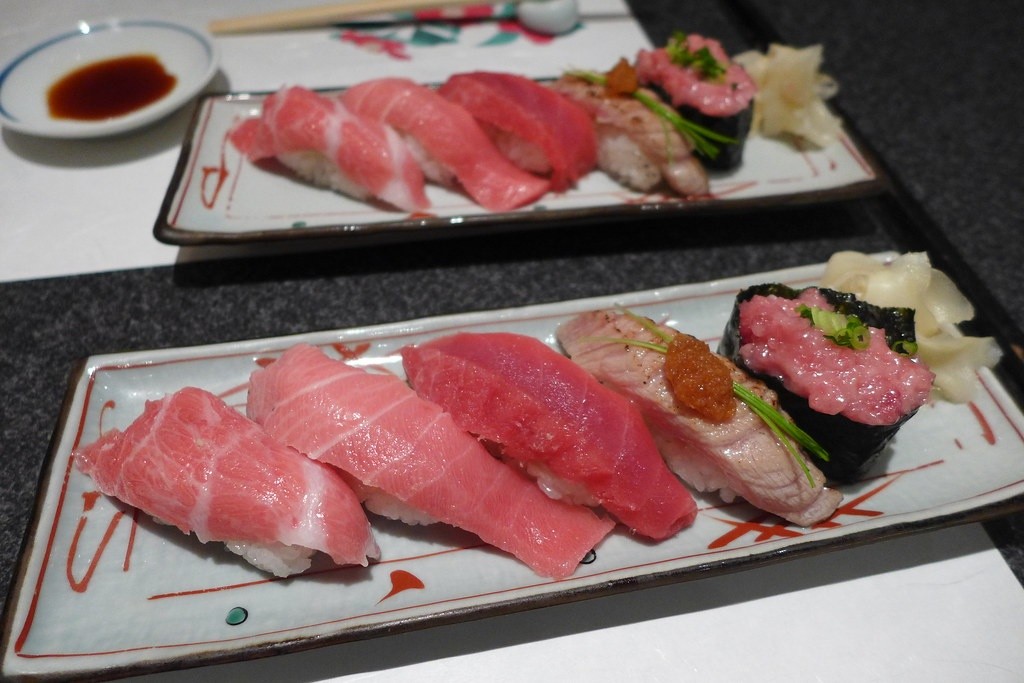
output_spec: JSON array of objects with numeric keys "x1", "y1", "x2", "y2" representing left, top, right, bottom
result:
[
  {"x1": 73, "y1": 281, "x2": 935, "y2": 578},
  {"x1": 231, "y1": 29, "x2": 756, "y2": 219}
]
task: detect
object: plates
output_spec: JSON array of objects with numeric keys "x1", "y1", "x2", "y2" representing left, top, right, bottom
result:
[
  {"x1": 0, "y1": 245, "x2": 1024, "y2": 683},
  {"x1": 1, "y1": 19, "x2": 221, "y2": 143},
  {"x1": 154, "y1": 59, "x2": 886, "y2": 241}
]
